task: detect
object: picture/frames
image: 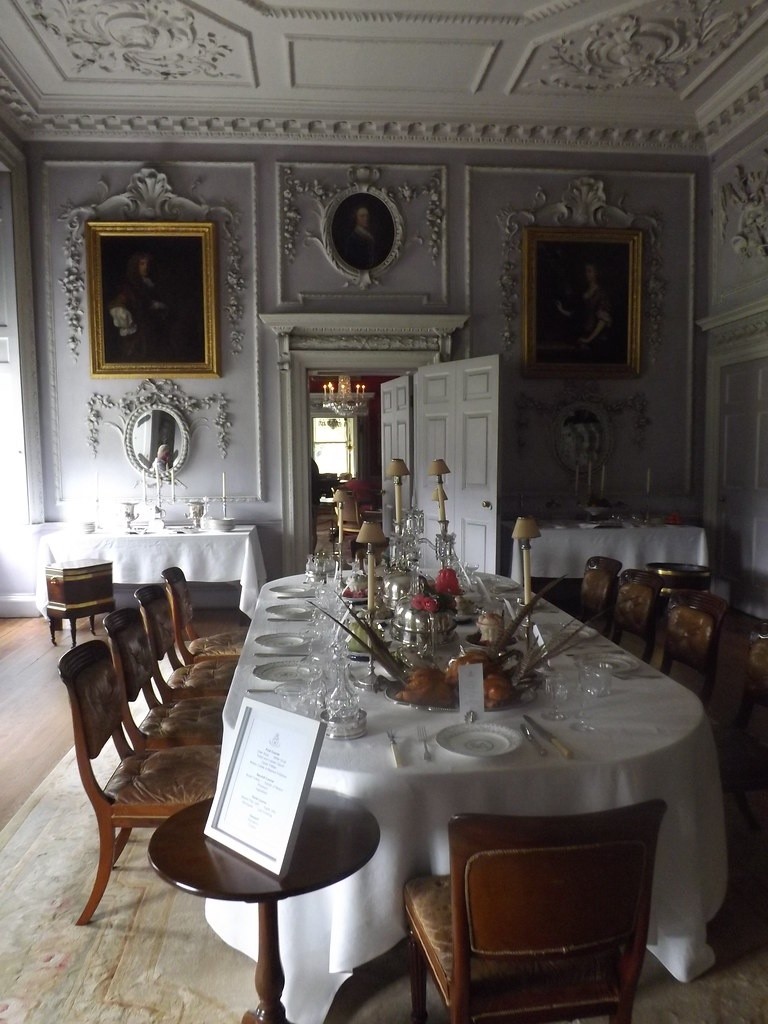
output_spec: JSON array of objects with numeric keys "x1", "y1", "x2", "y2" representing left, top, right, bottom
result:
[
  {"x1": 516, "y1": 226, "x2": 643, "y2": 379},
  {"x1": 83, "y1": 220, "x2": 219, "y2": 379},
  {"x1": 317, "y1": 185, "x2": 407, "y2": 290}
]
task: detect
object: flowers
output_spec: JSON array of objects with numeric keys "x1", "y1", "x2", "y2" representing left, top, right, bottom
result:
[
  {"x1": 343, "y1": 587, "x2": 368, "y2": 598},
  {"x1": 411, "y1": 569, "x2": 462, "y2": 614}
]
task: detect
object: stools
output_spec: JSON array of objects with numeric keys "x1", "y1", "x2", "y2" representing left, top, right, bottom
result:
[
  {"x1": 46, "y1": 559, "x2": 112, "y2": 648},
  {"x1": 646, "y1": 561, "x2": 711, "y2": 594}
]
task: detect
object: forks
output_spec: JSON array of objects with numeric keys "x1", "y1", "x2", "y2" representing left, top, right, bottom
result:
[{"x1": 417, "y1": 725, "x2": 432, "y2": 761}]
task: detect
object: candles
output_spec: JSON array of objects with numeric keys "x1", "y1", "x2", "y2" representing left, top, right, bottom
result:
[
  {"x1": 171, "y1": 468, "x2": 175, "y2": 501},
  {"x1": 646, "y1": 469, "x2": 652, "y2": 492},
  {"x1": 575, "y1": 465, "x2": 581, "y2": 493},
  {"x1": 144, "y1": 469, "x2": 147, "y2": 499},
  {"x1": 600, "y1": 465, "x2": 606, "y2": 488},
  {"x1": 222, "y1": 473, "x2": 227, "y2": 497},
  {"x1": 157, "y1": 466, "x2": 162, "y2": 497},
  {"x1": 588, "y1": 460, "x2": 593, "y2": 486}
]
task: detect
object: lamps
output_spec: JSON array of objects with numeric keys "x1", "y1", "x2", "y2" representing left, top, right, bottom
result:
[
  {"x1": 319, "y1": 418, "x2": 342, "y2": 430},
  {"x1": 332, "y1": 489, "x2": 350, "y2": 545},
  {"x1": 355, "y1": 520, "x2": 386, "y2": 610},
  {"x1": 511, "y1": 515, "x2": 542, "y2": 606},
  {"x1": 386, "y1": 459, "x2": 412, "y2": 525},
  {"x1": 425, "y1": 457, "x2": 452, "y2": 521},
  {"x1": 321, "y1": 375, "x2": 369, "y2": 419}
]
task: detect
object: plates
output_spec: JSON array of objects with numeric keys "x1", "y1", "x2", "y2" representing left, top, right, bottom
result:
[
  {"x1": 269, "y1": 584, "x2": 311, "y2": 594},
  {"x1": 490, "y1": 579, "x2": 520, "y2": 591},
  {"x1": 581, "y1": 653, "x2": 640, "y2": 673},
  {"x1": 265, "y1": 604, "x2": 311, "y2": 618},
  {"x1": 253, "y1": 633, "x2": 311, "y2": 651},
  {"x1": 252, "y1": 661, "x2": 311, "y2": 682},
  {"x1": 434, "y1": 722, "x2": 522, "y2": 755},
  {"x1": 554, "y1": 623, "x2": 599, "y2": 642}
]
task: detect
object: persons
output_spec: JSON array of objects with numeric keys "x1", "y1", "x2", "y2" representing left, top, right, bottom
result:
[
  {"x1": 310, "y1": 457, "x2": 322, "y2": 554},
  {"x1": 150, "y1": 444, "x2": 172, "y2": 470}
]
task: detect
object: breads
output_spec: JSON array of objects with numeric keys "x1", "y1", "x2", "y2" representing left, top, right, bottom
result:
[{"x1": 398, "y1": 649, "x2": 514, "y2": 709}]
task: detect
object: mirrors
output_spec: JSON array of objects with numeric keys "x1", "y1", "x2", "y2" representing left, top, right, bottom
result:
[
  {"x1": 127, "y1": 405, "x2": 190, "y2": 473},
  {"x1": 555, "y1": 402, "x2": 615, "y2": 476}
]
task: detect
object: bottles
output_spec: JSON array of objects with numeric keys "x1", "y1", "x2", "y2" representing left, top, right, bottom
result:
[{"x1": 200, "y1": 497, "x2": 213, "y2": 531}]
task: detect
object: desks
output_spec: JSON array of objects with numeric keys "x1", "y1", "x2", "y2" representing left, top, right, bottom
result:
[
  {"x1": 508, "y1": 520, "x2": 707, "y2": 593},
  {"x1": 191, "y1": 556, "x2": 725, "y2": 1024},
  {"x1": 145, "y1": 787, "x2": 382, "y2": 1024},
  {"x1": 31, "y1": 521, "x2": 267, "y2": 620}
]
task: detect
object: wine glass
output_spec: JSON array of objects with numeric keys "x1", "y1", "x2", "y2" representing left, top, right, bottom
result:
[{"x1": 274, "y1": 551, "x2": 613, "y2": 741}]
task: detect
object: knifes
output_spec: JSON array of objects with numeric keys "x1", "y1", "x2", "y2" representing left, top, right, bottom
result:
[{"x1": 522, "y1": 714, "x2": 573, "y2": 758}]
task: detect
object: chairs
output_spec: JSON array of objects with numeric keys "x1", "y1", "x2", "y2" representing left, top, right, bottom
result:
[
  {"x1": 712, "y1": 620, "x2": 768, "y2": 833},
  {"x1": 58, "y1": 638, "x2": 224, "y2": 929},
  {"x1": 133, "y1": 584, "x2": 241, "y2": 696},
  {"x1": 400, "y1": 800, "x2": 671, "y2": 1024},
  {"x1": 610, "y1": 569, "x2": 667, "y2": 664},
  {"x1": 161, "y1": 567, "x2": 250, "y2": 666},
  {"x1": 575, "y1": 556, "x2": 624, "y2": 637},
  {"x1": 658, "y1": 588, "x2": 728, "y2": 705},
  {"x1": 335, "y1": 491, "x2": 363, "y2": 533},
  {"x1": 102, "y1": 607, "x2": 230, "y2": 747}
]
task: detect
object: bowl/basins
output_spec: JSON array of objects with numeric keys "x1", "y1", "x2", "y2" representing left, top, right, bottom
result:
[
  {"x1": 211, "y1": 518, "x2": 236, "y2": 532},
  {"x1": 81, "y1": 522, "x2": 95, "y2": 533}
]
